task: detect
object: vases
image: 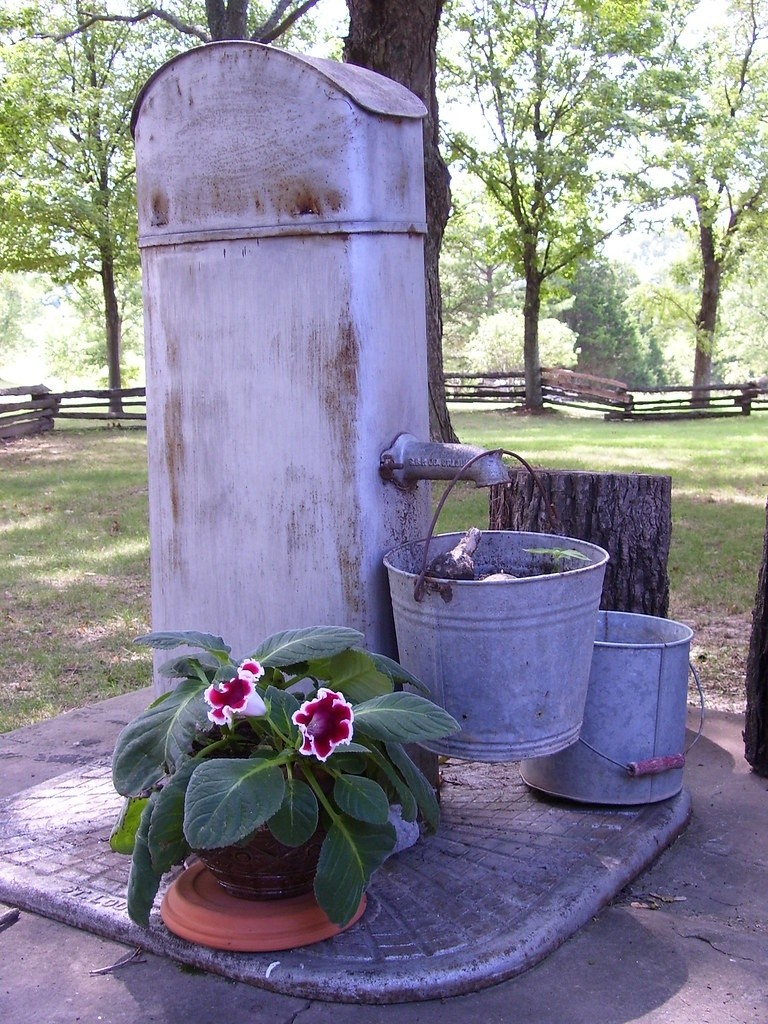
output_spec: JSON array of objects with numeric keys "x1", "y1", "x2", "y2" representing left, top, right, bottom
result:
[{"x1": 184, "y1": 827, "x2": 322, "y2": 902}]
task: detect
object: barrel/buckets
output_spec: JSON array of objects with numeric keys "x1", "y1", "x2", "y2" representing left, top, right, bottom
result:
[
  {"x1": 384, "y1": 449, "x2": 609, "y2": 763},
  {"x1": 519, "y1": 610, "x2": 703, "y2": 805}
]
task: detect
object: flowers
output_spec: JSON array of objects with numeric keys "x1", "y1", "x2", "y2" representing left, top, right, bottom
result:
[{"x1": 106, "y1": 624, "x2": 459, "y2": 933}]
